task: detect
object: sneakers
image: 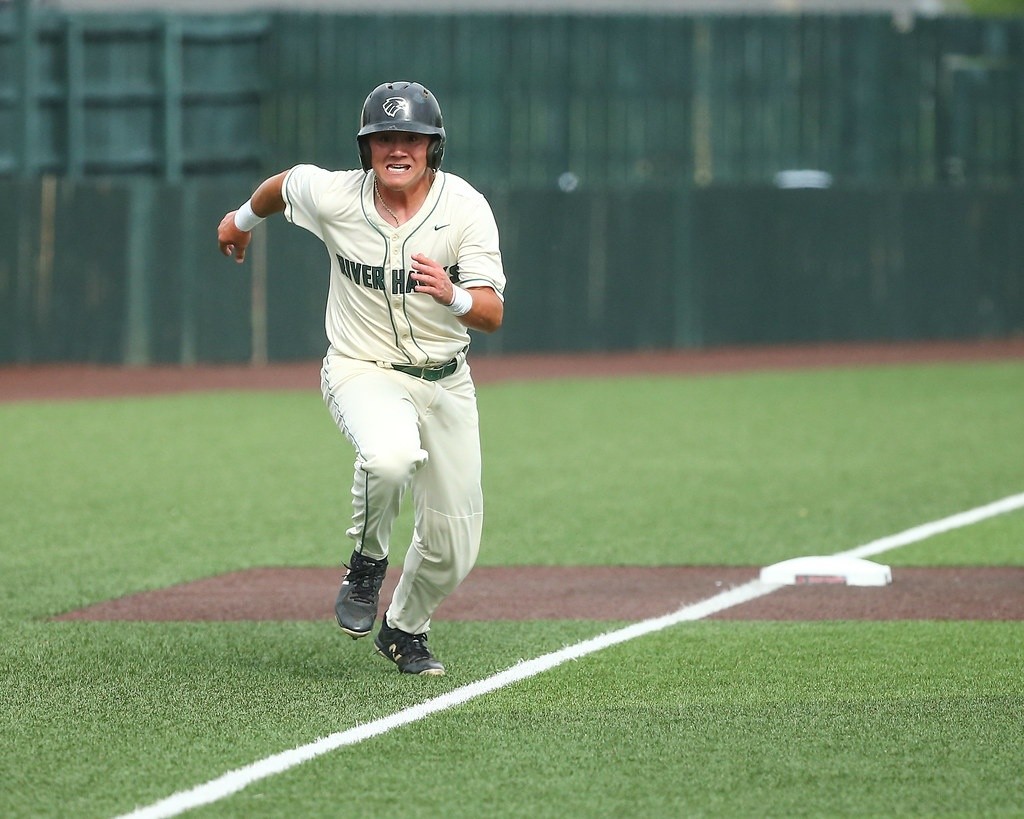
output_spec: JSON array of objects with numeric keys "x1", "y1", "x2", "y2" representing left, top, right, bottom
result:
[
  {"x1": 374, "y1": 611, "x2": 445, "y2": 675},
  {"x1": 334, "y1": 549, "x2": 390, "y2": 640}
]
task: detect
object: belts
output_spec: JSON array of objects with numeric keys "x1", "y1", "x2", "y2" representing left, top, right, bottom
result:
[{"x1": 395, "y1": 361, "x2": 457, "y2": 381}]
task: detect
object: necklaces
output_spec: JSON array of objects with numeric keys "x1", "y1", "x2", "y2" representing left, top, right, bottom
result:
[{"x1": 374, "y1": 177, "x2": 399, "y2": 226}]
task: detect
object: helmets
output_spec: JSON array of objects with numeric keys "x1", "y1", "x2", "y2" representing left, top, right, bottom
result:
[{"x1": 357, "y1": 81, "x2": 446, "y2": 177}]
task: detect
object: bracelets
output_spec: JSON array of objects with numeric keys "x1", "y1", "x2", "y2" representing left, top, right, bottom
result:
[
  {"x1": 235, "y1": 199, "x2": 268, "y2": 232},
  {"x1": 444, "y1": 284, "x2": 473, "y2": 316}
]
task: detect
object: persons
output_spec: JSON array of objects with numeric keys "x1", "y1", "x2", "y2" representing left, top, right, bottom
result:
[{"x1": 217, "y1": 82, "x2": 506, "y2": 674}]
